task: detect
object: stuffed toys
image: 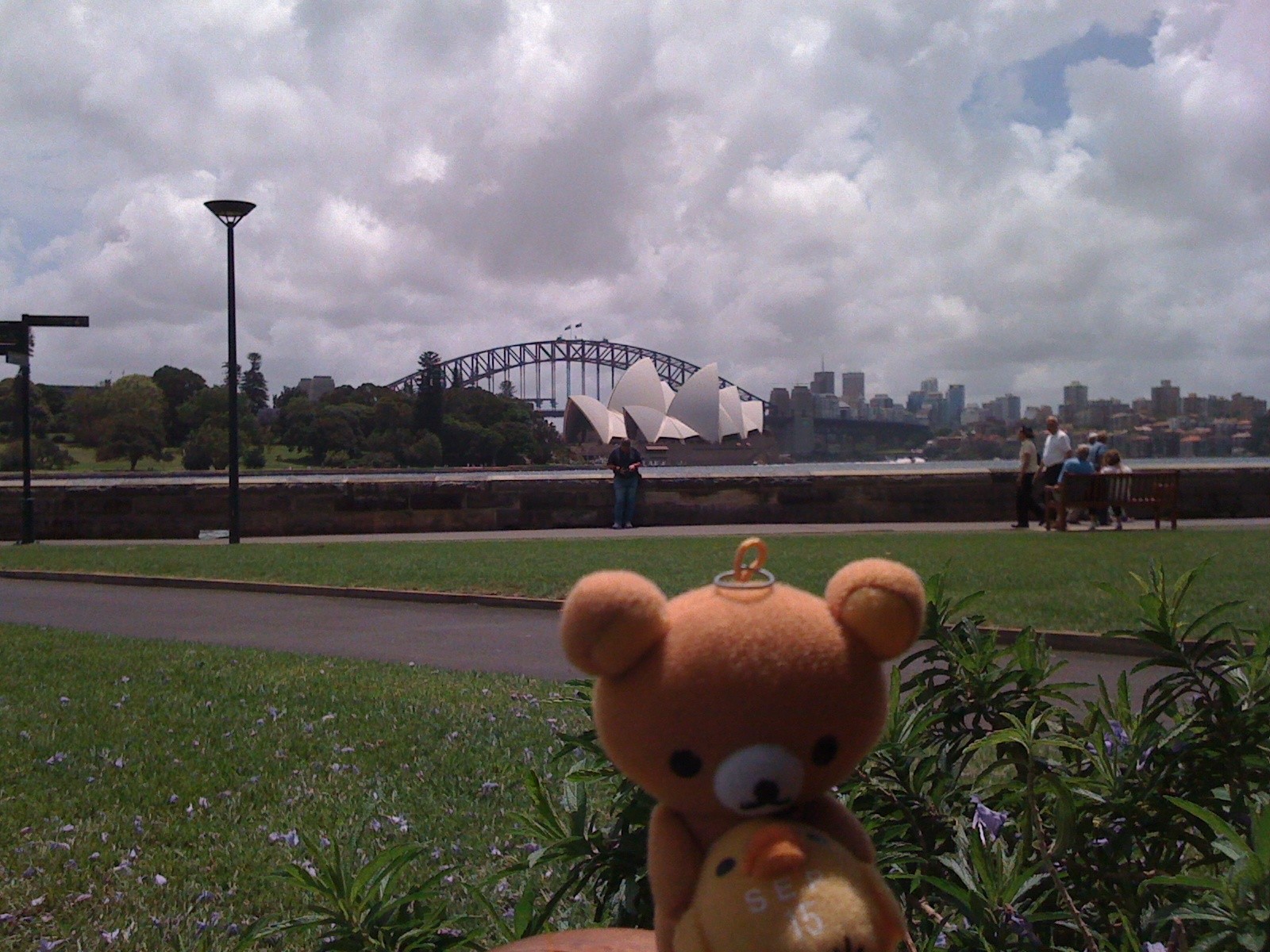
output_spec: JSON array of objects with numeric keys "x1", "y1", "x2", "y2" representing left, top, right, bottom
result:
[
  {"x1": 562, "y1": 538, "x2": 925, "y2": 952},
  {"x1": 672, "y1": 820, "x2": 906, "y2": 952}
]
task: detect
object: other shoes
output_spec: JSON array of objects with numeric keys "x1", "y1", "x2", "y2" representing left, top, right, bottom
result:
[
  {"x1": 1116, "y1": 525, "x2": 1122, "y2": 530},
  {"x1": 1011, "y1": 522, "x2": 1029, "y2": 528},
  {"x1": 1052, "y1": 523, "x2": 1065, "y2": 530},
  {"x1": 1039, "y1": 519, "x2": 1044, "y2": 525},
  {"x1": 1100, "y1": 518, "x2": 1112, "y2": 526},
  {"x1": 1087, "y1": 526, "x2": 1094, "y2": 531}
]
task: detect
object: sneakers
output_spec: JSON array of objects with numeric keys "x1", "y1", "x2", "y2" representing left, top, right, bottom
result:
[
  {"x1": 612, "y1": 522, "x2": 622, "y2": 529},
  {"x1": 625, "y1": 522, "x2": 633, "y2": 529}
]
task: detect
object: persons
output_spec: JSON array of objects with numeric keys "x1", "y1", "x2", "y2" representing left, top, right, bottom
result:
[
  {"x1": 1044, "y1": 430, "x2": 1136, "y2": 530},
  {"x1": 607, "y1": 438, "x2": 643, "y2": 529},
  {"x1": 1032, "y1": 417, "x2": 1072, "y2": 527},
  {"x1": 1011, "y1": 426, "x2": 1049, "y2": 528}
]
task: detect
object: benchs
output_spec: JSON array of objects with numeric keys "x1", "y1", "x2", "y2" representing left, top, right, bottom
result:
[{"x1": 1044, "y1": 468, "x2": 1181, "y2": 532}]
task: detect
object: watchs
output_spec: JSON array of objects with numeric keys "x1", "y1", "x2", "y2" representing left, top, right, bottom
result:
[{"x1": 614, "y1": 465, "x2": 620, "y2": 472}]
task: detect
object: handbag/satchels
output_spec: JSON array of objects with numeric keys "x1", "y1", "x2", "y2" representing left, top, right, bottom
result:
[{"x1": 1037, "y1": 454, "x2": 1041, "y2": 465}]
